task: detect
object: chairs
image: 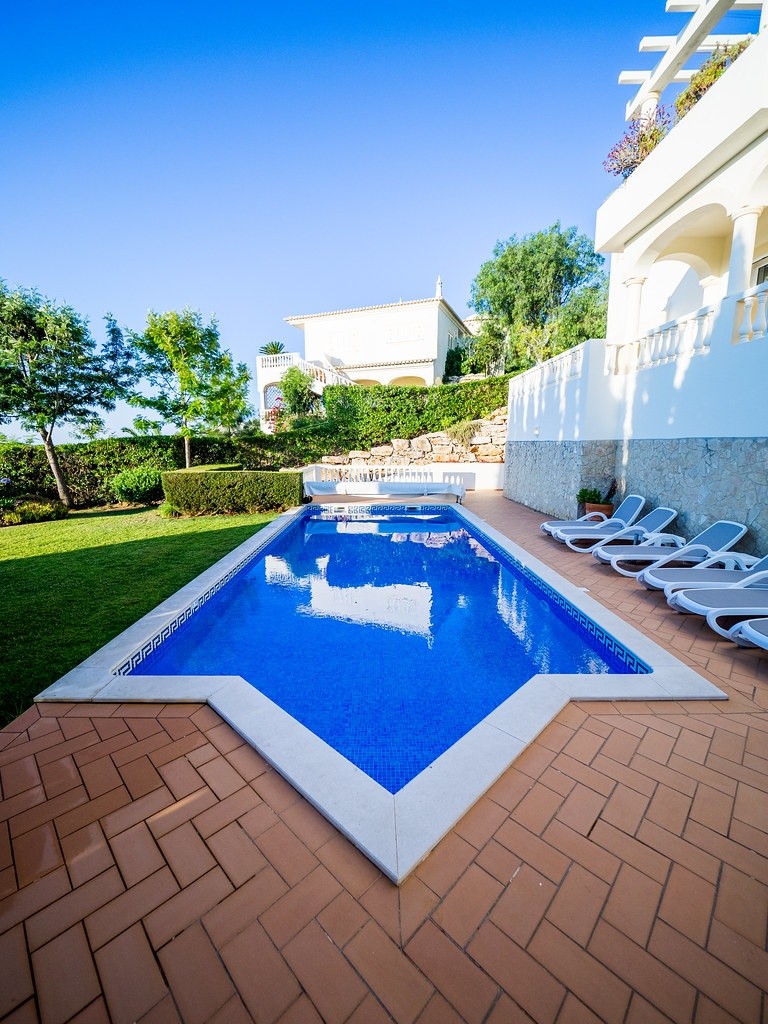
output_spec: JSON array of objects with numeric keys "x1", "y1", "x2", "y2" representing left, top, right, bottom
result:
[
  {"x1": 554, "y1": 507, "x2": 679, "y2": 553},
  {"x1": 611, "y1": 555, "x2": 768, "y2": 674},
  {"x1": 592, "y1": 520, "x2": 749, "y2": 577},
  {"x1": 540, "y1": 494, "x2": 647, "y2": 537}
]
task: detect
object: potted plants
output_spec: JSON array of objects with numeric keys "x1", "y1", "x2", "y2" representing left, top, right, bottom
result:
[{"x1": 575, "y1": 478, "x2": 617, "y2": 522}]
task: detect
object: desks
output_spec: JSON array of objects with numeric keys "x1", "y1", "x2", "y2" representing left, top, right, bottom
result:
[
  {"x1": 706, "y1": 552, "x2": 761, "y2": 570},
  {"x1": 642, "y1": 533, "x2": 687, "y2": 565}
]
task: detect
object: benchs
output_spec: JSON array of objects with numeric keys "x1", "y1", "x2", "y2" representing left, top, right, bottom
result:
[{"x1": 303, "y1": 481, "x2": 466, "y2": 505}]
